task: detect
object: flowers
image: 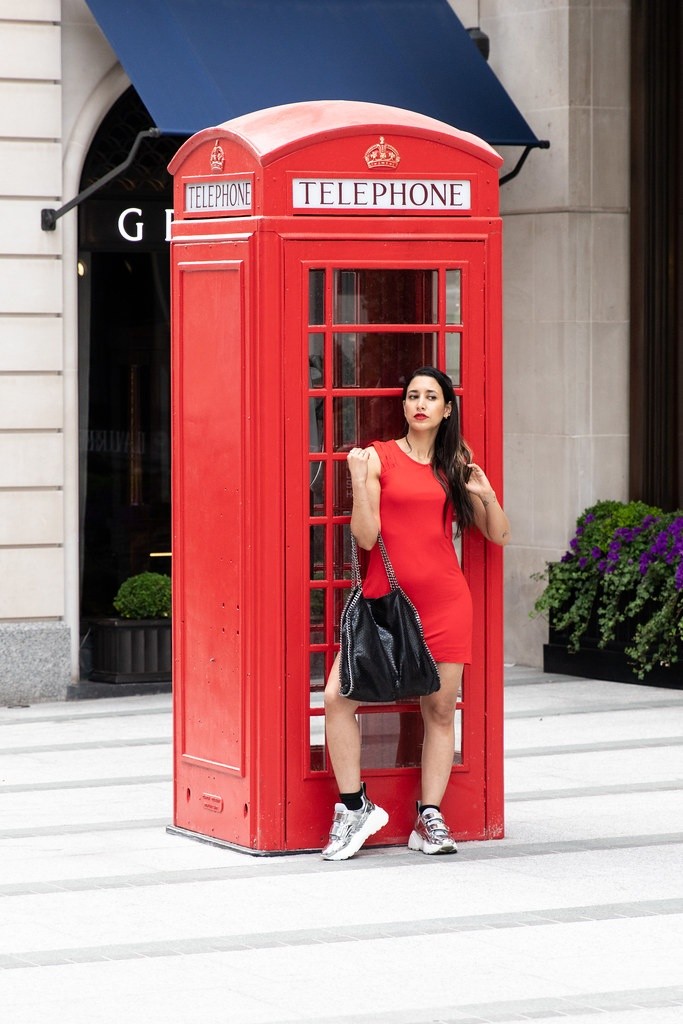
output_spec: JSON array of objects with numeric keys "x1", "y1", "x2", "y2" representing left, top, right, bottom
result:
[{"x1": 531, "y1": 502, "x2": 683, "y2": 680}]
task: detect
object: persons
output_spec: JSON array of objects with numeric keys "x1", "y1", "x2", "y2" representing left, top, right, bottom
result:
[{"x1": 320, "y1": 366, "x2": 512, "y2": 861}]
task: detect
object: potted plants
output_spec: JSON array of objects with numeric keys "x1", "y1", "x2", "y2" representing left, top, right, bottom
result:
[{"x1": 94, "y1": 570, "x2": 171, "y2": 684}]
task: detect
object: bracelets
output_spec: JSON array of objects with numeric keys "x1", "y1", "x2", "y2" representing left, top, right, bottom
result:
[
  {"x1": 353, "y1": 493, "x2": 369, "y2": 505},
  {"x1": 483, "y1": 495, "x2": 496, "y2": 506}
]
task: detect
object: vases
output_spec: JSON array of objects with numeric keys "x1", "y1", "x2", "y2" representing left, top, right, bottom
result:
[{"x1": 541, "y1": 581, "x2": 683, "y2": 691}]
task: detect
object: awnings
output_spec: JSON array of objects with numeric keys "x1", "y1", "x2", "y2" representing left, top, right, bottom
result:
[{"x1": 41, "y1": 0, "x2": 550, "y2": 232}]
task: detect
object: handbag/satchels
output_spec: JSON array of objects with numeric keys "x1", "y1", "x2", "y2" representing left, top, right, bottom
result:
[{"x1": 338, "y1": 528, "x2": 439, "y2": 703}]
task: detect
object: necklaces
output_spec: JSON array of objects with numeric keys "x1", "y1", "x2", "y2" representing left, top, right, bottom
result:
[{"x1": 412, "y1": 451, "x2": 426, "y2": 459}]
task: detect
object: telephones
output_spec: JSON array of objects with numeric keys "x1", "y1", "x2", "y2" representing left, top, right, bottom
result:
[{"x1": 309, "y1": 354, "x2": 326, "y2": 419}]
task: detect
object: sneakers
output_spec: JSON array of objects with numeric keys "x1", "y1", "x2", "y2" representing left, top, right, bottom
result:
[
  {"x1": 409, "y1": 800, "x2": 458, "y2": 854},
  {"x1": 320, "y1": 783, "x2": 389, "y2": 861}
]
